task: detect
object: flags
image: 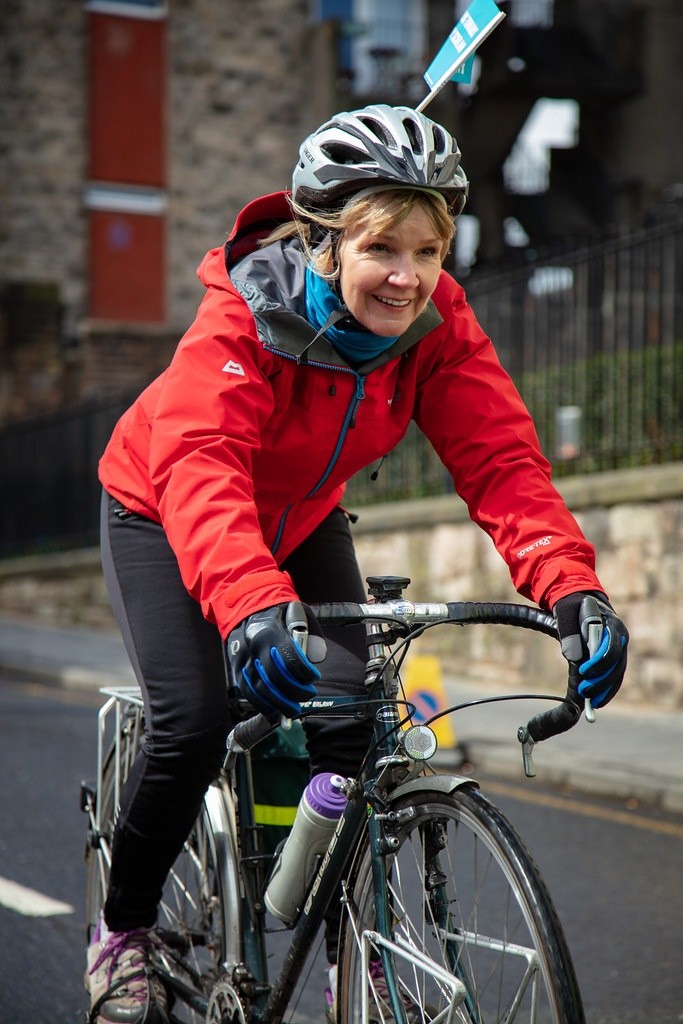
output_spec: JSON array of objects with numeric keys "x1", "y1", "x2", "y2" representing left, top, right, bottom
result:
[{"x1": 423, "y1": 0, "x2": 502, "y2": 90}]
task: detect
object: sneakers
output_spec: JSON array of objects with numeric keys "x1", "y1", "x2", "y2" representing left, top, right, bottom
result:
[
  {"x1": 324, "y1": 956, "x2": 444, "y2": 1024},
  {"x1": 87, "y1": 911, "x2": 167, "y2": 1024}
]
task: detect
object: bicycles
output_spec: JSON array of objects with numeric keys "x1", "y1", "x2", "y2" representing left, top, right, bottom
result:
[{"x1": 79, "y1": 574, "x2": 606, "y2": 1024}]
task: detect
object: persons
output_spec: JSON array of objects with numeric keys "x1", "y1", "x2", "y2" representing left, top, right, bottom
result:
[{"x1": 83, "y1": 106, "x2": 630, "y2": 1024}]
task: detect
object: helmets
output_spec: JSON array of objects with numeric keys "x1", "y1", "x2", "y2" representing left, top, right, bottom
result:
[{"x1": 290, "y1": 103, "x2": 470, "y2": 224}]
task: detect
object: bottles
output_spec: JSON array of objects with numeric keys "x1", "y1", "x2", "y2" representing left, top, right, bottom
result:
[{"x1": 264, "y1": 773, "x2": 354, "y2": 922}]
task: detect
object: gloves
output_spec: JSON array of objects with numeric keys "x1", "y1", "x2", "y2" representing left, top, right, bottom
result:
[
  {"x1": 225, "y1": 599, "x2": 327, "y2": 728},
  {"x1": 552, "y1": 589, "x2": 630, "y2": 710}
]
